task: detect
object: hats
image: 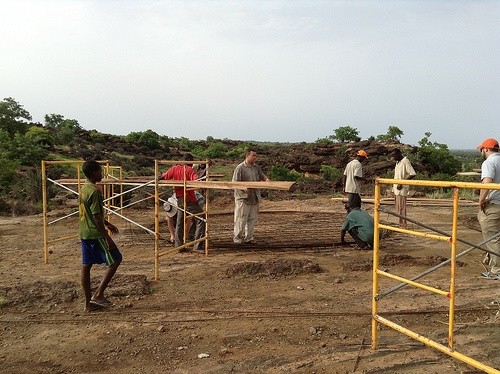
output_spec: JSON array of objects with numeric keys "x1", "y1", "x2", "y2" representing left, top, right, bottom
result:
[
  {"x1": 163, "y1": 198, "x2": 178, "y2": 218},
  {"x1": 476, "y1": 138, "x2": 499, "y2": 150},
  {"x1": 357, "y1": 149, "x2": 368, "y2": 159}
]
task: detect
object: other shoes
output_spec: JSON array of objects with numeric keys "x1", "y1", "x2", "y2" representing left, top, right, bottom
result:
[
  {"x1": 193, "y1": 249, "x2": 205, "y2": 253},
  {"x1": 481, "y1": 272, "x2": 499, "y2": 280}
]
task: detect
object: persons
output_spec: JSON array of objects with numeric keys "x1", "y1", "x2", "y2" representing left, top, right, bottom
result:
[
  {"x1": 342, "y1": 150, "x2": 372, "y2": 214},
  {"x1": 476, "y1": 139, "x2": 500, "y2": 281},
  {"x1": 78, "y1": 159, "x2": 123, "y2": 313},
  {"x1": 390, "y1": 148, "x2": 417, "y2": 226},
  {"x1": 232, "y1": 151, "x2": 272, "y2": 247},
  {"x1": 164, "y1": 153, "x2": 210, "y2": 254},
  {"x1": 341, "y1": 200, "x2": 383, "y2": 250}
]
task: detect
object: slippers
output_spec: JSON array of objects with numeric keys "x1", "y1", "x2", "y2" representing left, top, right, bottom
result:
[
  {"x1": 89, "y1": 300, "x2": 114, "y2": 308},
  {"x1": 85, "y1": 306, "x2": 103, "y2": 311}
]
